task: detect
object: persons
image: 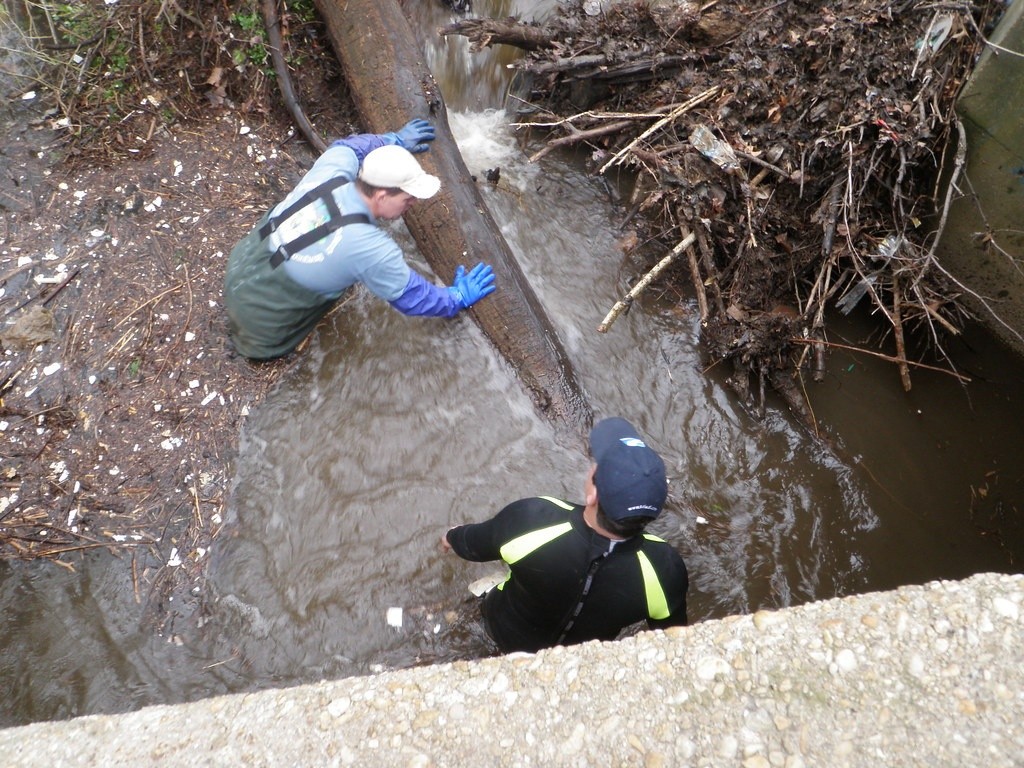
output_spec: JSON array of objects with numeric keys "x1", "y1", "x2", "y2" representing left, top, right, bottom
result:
[
  {"x1": 432, "y1": 417, "x2": 688, "y2": 654},
  {"x1": 223, "y1": 116, "x2": 496, "y2": 362}
]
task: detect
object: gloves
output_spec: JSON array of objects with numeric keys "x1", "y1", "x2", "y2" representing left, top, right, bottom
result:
[
  {"x1": 331, "y1": 118, "x2": 435, "y2": 164},
  {"x1": 387, "y1": 263, "x2": 496, "y2": 317}
]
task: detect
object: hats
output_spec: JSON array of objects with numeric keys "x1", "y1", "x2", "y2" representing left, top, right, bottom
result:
[
  {"x1": 590, "y1": 417, "x2": 668, "y2": 525},
  {"x1": 359, "y1": 145, "x2": 441, "y2": 200}
]
